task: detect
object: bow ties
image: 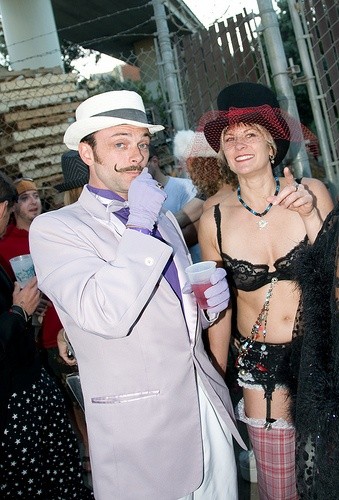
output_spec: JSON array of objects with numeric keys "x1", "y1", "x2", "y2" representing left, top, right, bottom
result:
[{"x1": 90, "y1": 192, "x2": 128, "y2": 213}]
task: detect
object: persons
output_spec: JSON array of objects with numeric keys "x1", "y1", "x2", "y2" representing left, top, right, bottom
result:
[
  {"x1": 140, "y1": 145, "x2": 201, "y2": 264},
  {"x1": 29, "y1": 90, "x2": 248, "y2": 500},
  {"x1": 172, "y1": 110, "x2": 238, "y2": 247},
  {"x1": 0, "y1": 172, "x2": 87, "y2": 500},
  {"x1": 0, "y1": 175, "x2": 76, "y2": 374},
  {"x1": 199, "y1": 82, "x2": 339, "y2": 500},
  {"x1": 57, "y1": 150, "x2": 92, "y2": 366}
]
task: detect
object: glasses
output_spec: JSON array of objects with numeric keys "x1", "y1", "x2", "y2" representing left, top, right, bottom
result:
[{"x1": 13, "y1": 178, "x2": 33, "y2": 184}]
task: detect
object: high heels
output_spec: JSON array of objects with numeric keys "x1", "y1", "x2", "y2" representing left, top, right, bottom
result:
[{"x1": 239, "y1": 449, "x2": 257, "y2": 483}]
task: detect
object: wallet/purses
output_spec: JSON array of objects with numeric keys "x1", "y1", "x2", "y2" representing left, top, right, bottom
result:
[{"x1": 65, "y1": 372, "x2": 86, "y2": 413}]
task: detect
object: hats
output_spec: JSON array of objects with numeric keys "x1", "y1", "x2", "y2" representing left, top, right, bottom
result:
[
  {"x1": 63, "y1": 90, "x2": 166, "y2": 151},
  {"x1": 14, "y1": 179, "x2": 37, "y2": 195},
  {"x1": 54, "y1": 149, "x2": 90, "y2": 194},
  {"x1": 204, "y1": 82, "x2": 291, "y2": 169}
]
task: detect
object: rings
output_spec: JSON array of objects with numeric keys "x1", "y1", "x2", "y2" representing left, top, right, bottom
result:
[
  {"x1": 156, "y1": 183, "x2": 165, "y2": 190},
  {"x1": 293, "y1": 182, "x2": 300, "y2": 192}
]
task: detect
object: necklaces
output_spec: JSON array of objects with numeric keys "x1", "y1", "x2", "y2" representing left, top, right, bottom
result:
[{"x1": 237, "y1": 176, "x2": 280, "y2": 228}]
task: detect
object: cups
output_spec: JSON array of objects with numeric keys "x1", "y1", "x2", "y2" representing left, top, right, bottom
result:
[
  {"x1": 9, "y1": 254, "x2": 35, "y2": 289},
  {"x1": 185, "y1": 260, "x2": 217, "y2": 309}
]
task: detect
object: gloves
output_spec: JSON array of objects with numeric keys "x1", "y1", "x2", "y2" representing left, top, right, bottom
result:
[
  {"x1": 126, "y1": 167, "x2": 168, "y2": 232},
  {"x1": 182, "y1": 268, "x2": 231, "y2": 316}
]
